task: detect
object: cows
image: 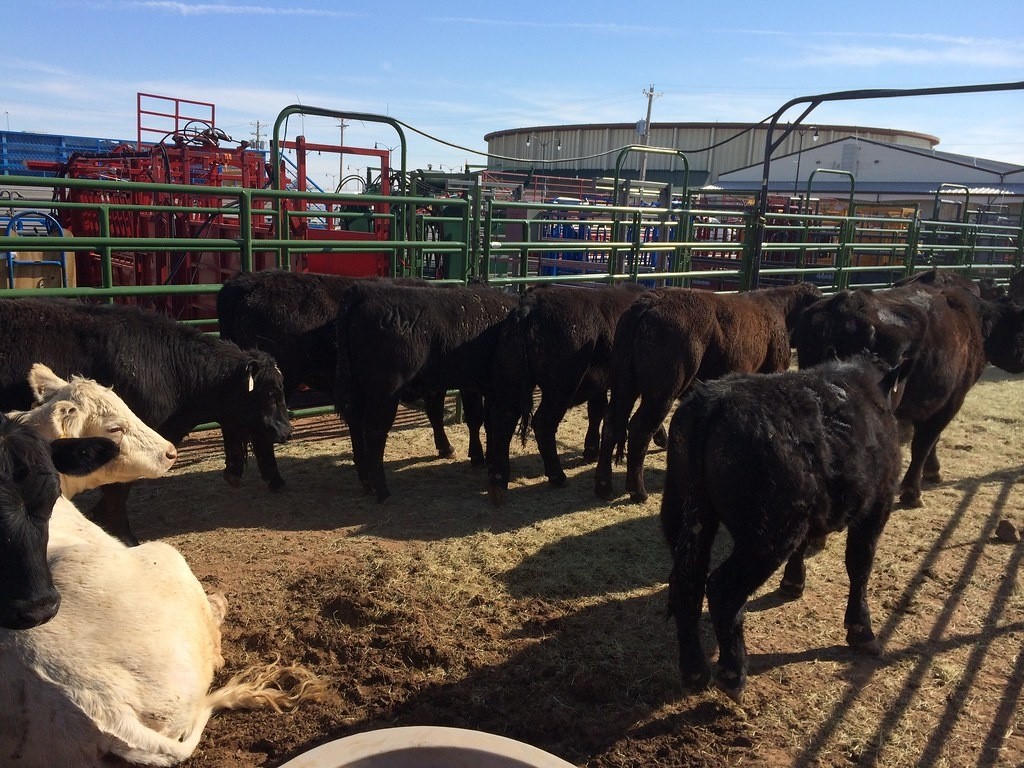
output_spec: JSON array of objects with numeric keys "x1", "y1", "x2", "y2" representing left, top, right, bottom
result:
[
  {"x1": 484, "y1": 285, "x2": 668, "y2": 504},
  {"x1": 660, "y1": 350, "x2": 918, "y2": 706},
  {"x1": 0, "y1": 364, "x2": 334, "y2": 768},
  {"x1": 788, "y1": 272, "x2": 1024, "y2": 509},
  {"x1": 595, "y1": 282, "x2": 828, "y2": 502},
  {"x1": 216, "y1": 272, "x2": 522, "y2": 499},
  {"x1": 0, "y1": 296, "x2": 292, "y2": 547}
]
